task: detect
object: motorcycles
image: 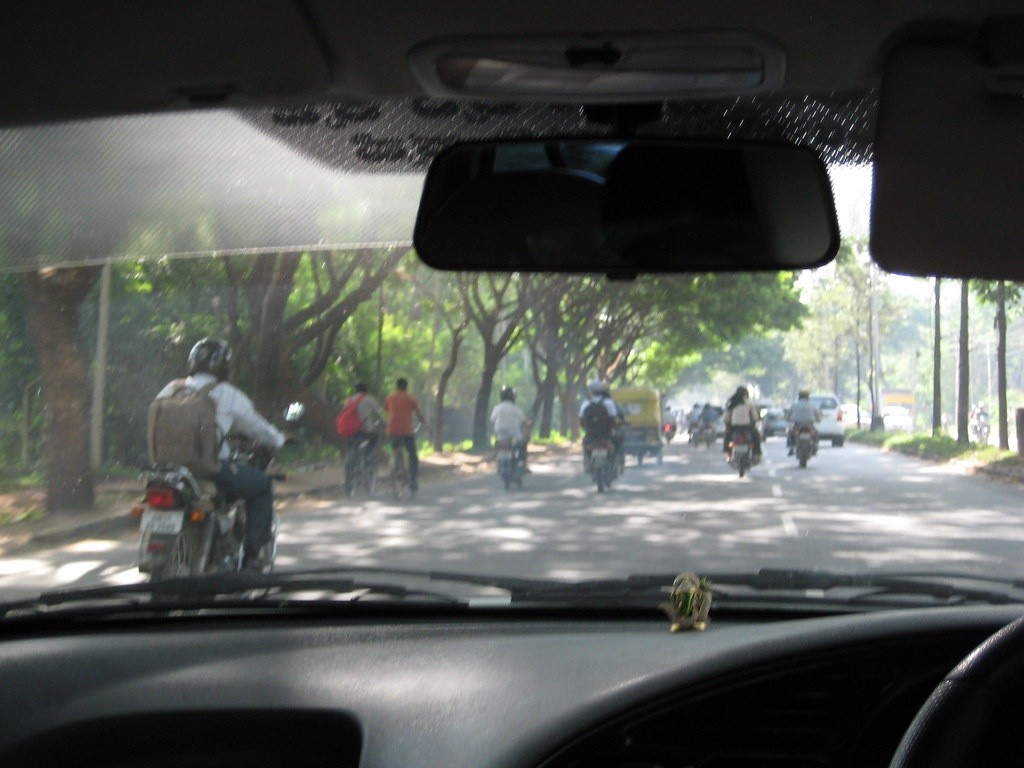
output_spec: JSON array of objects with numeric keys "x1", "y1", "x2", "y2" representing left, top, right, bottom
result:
[
  {"x1": 131, "y1": 400, "x2": 306, "y2": 617},
  {"x1": 782, "y1": 409, "x2": 824, "y2": 467},
  {"x1": 727, "y1": 407, "x2": 768, "y2": 478},
  {"x1": 491, "y1": 420, "x2": 534, "y2": 492},
  {"x1": 587, "y1": 421, "x2": 637, "y2": 492},
  {"x1": 689, "y1": 419, "x2": 717, "y2": 448},
  {"x1": 609, "y1": 388, "x2": 671, "y2": 469},
  {"x1": 972, "y1": 414, "x2": 993, "y2": 445},
  {"x1": 661, "y1": 423, "x2": 675, "y2": 444}
]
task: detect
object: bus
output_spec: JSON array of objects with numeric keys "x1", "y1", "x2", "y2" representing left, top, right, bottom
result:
[{"x1": 869, "y1": 387, "x2": 914, "y2": 418}]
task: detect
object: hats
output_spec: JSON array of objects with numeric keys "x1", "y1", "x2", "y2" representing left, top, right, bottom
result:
[{"x1": 587, "y1": 380, "x2": 605, "y2": 392}]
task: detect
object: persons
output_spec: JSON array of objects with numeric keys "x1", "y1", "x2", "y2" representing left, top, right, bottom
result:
[
  {"x1": 660, "y1": 403, "x2": 723, "y2": 444},
  {"x1": 579, "y1": 379, "x2": 630, "y2": 474},
  {"x1": 968, "y1": 401, "x2": 990, "y2": 435},
  {"x1": 384, "y1": 378, "x2": 426, "y2": 491},
  {"x1": 149, "y1": 334, "x2": 294, "y2": 572},
  {"x1": 723, "y1": 386, "x2": 764, "y2": 464},
  {"x1": 786, "y1": 390, "x2": 821, "y2": 457},
  {"x1": 337, "y1": 381, "x2": 385, "y2": 497},
  {"x1": 489, "y1": 386, "x2": 533, "y2": 476}
]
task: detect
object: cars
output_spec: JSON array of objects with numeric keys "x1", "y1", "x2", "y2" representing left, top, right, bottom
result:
[
  {"x1": 795, "y1": 394, "x2": 846, "y2": 448},
  {"x1": 761, "y1": 409, "x2": 788, "y2": 438}
]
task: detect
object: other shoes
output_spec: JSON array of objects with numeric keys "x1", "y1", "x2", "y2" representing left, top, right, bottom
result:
[
  {"x1": 787, "y1": 449, "x2": 794, "y2": 456},
  {"x1": 410, "y1": 481, "x2": 419, "y2": 492},
  {"x1": 241, "y1": 557, "x2": 264, "y2": 571}
]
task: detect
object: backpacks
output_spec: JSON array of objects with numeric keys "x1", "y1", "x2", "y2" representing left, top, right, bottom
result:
[
  {"x1": 583, "y1": 397, "x2": 610, "y2": 438},
  {"x1": 147, "y1": 377, "x2": 226, "y2": 480},
  {"x1": 336, "y1": 392, "x2": 363, "y2": 438}
]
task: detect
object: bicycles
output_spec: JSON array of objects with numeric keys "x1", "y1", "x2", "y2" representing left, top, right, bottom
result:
[
  {"x1": 388, "y1": 422, "x2": 424, "y2": 505},
  {"x1": 343, "y1": 420, "x2": 383, "y2": 501}
]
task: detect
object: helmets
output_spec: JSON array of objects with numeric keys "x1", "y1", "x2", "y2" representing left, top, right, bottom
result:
[
  {"x1": 499, "y1": 384, "x2": 517, "y2": 399},
  {"x1": 797, "y1": 387, "x2": 809, "y2": 397},
  {"x1": 185, "y1": 336, "x2": 232, "y2": 380}
]
